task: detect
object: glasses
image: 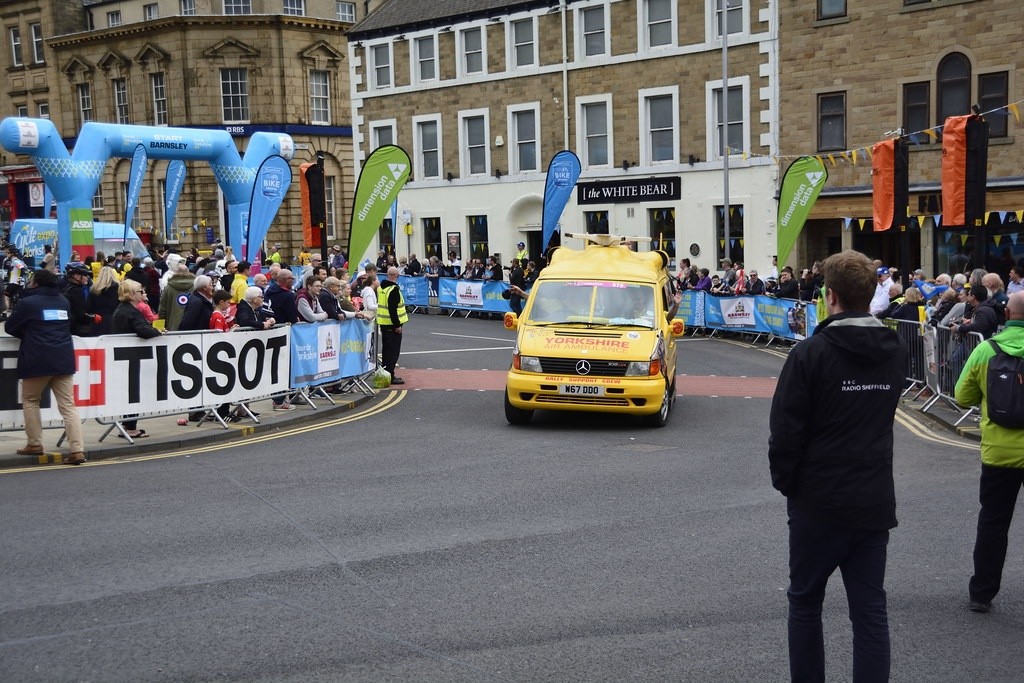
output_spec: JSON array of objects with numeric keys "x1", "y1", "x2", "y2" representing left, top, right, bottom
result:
[
  {"x1": 750, "y1": 275, "x2": 756, "y2": 277},
  {"x1": 132, "y1": 290, "x2": 145, "y2": 295}
]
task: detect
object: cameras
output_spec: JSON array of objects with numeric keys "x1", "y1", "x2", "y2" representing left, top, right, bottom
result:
[
  {"x1": 800, "y1": 269, "x2": 813, "y2": 274},
  {"x1": 949, "y1": 319, "x2": 963, "y2": 328},
  {"x1": 504, "y1": 266, "x2": 513, "y2": 272}
]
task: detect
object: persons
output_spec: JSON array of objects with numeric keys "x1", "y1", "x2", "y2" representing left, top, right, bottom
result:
[
  {"x1": 868, "y1": 259, "x2": 1024, "y2": 422},
  {"x1": 675, "y1": 258, "x2": 827, "y2": 352},
  {"x1": 954, "y1": 290, "x2": 1024, "y2": 612},
  {"x1": 377, "y1": 267, "x2": 408, "y2": 385},
  {"x1": 621, "y1": 289, "x2": 682, "y2": 323},
  {"x1": 769, "y1": 250, "x2": 908, "y2": 683},
  {"x1": 0, "y1": 230, "x2": 380, "y2": 438},
  {"x1": 376, "y1": 242, "x2": 540, "y2": 319},
  {"x1": 4, "y1": 269, "x2": 85, "y2": 465},
  {"x1": 509, "y1": 285, "x2": 579, "y2": 317}
]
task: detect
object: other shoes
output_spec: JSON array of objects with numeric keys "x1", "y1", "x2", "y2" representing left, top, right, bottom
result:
[
  {"x1": 238, "y1": 413, "x2": 251, "y2": 418},
  {"x1": 410, "y1": 305, "x2": 504, "y2": 321},
  {"x1": 391, "y1": 377, "x2": 405, "y2": 384},
  {"x1": 272, "y1": 387, "x2": 346, "y2": 411},
  {"x1": 968, "y1": 582, "x2": 994, "y2": 613},
  {"x1": 248, "y1": 411, "x2": 262, "y2": 417}
]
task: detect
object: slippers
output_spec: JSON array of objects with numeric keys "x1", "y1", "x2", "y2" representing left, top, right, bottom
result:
[{"x1": 118, "y1": 428, "x2": 149, "y2": 438}]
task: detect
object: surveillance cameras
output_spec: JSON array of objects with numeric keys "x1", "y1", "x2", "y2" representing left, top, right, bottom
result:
[{"x1": 884, "y1": 131, "x2": 894, "y2": 138}]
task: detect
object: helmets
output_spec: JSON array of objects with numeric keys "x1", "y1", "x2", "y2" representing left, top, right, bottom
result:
[
  {"x1": 66, "y1": 263, "x2": 91, "y2": 279},
  {"x1": 502, "y1": 289, "x2": 512, "y2": 300},
  {"x1": 204, "y1": 271, "x2": 220, "y2": 277}
]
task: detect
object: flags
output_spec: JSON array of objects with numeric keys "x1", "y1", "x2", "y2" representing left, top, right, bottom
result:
[{"x1": 914, "y1": 280, "x2": 949, "y2": 300}]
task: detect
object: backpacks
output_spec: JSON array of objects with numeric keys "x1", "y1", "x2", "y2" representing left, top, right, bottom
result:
[{"x1": 987, "y1": 337, "x2": 1024, "y2": 429}]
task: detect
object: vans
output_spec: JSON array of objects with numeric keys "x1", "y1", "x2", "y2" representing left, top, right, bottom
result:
[
  {"x1": 8, "y1": 218, "x2": 153, "y2": 279},
  {"x1": 503, "y1": 233, "x2": 687, "y2": 429}
]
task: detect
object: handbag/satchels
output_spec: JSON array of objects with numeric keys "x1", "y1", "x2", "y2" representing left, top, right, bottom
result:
[{"x1": 371, "y1": 365, "x2": 391, "y2": 388}]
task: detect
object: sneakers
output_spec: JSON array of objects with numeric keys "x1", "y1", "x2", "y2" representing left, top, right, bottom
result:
[
  {"x1": 188, "y1": 412, "x2": 217, "y2": 422},
  {"x1": 15, "y1": 444, "x2": 44, "y2": 456},
  {"x1": 62, "y1": 453, "x2": 86, "y2": 465},
  {"x1": 214, "y1": 413, "x2": 242, "y2": 423}
]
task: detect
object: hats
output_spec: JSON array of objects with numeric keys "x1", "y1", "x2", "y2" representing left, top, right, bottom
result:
[
  {"x1": 913, "y1": 268, "x2": 926, "y2": 275},
  {"x1": 719, "y1": 257, "x2": 731, "y2": 263},
  {"x1": 333, "y1": 244, "x2": 340, "y2": 251},
  {"x1": 447, "y1": 251, "x2": 456, "y2": 256},
  {"x1": 875, "y1": 266, "x2": 889, "y2": 275},
  {"x1": 517, "y1": 242, "x2": 524, "y2": 246}
]
